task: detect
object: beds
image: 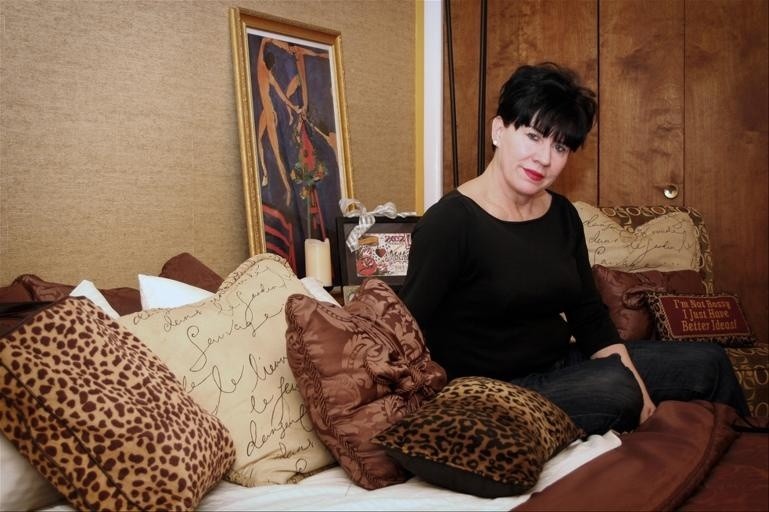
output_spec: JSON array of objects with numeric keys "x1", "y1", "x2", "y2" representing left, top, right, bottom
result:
[{"x1": 0, "y1": 253, "x2": 769, "y2": 511}]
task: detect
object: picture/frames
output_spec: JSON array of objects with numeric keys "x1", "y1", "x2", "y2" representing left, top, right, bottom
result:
[
  {"x1": 229, "y1": 3, "x2": 358, "y2": 289},
  {"x1": 335, "y1": 216, "x2": 422, "y2": 291}
]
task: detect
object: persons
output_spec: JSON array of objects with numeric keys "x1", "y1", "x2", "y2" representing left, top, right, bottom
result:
[{"x1": 398, "y1": 62, "x2": 752, "y2": 439}]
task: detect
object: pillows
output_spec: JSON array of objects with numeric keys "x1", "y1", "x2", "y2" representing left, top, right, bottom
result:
[
  {"x1": 118, "y1": 252, "x2": 340, "y2": 487},
  {"x1": 136, "y1": 273, "x2": 343, "y2": 310},
  {"x1": 376, "y1": 372, "x2": 578, "y2": 500},
  {"x1": 572, "y1": 200, "x2": 757, "y2": 346},
  {"x1": 284, "y1": 278, "x2": 448, "y2": 489}
]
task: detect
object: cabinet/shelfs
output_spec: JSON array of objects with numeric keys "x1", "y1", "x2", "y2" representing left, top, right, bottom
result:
[{"x1": 443, "y1": 0, "x2": 767, "y2": 345}]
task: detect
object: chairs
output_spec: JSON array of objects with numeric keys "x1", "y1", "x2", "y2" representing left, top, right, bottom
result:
[{"x1": 573, "y1": 206, "x2": 769, "y2": 418}]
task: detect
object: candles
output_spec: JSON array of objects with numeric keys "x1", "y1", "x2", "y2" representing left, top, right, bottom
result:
[{"x1": 304, "y1": 237, "x2": 333, "y2": 287}]
